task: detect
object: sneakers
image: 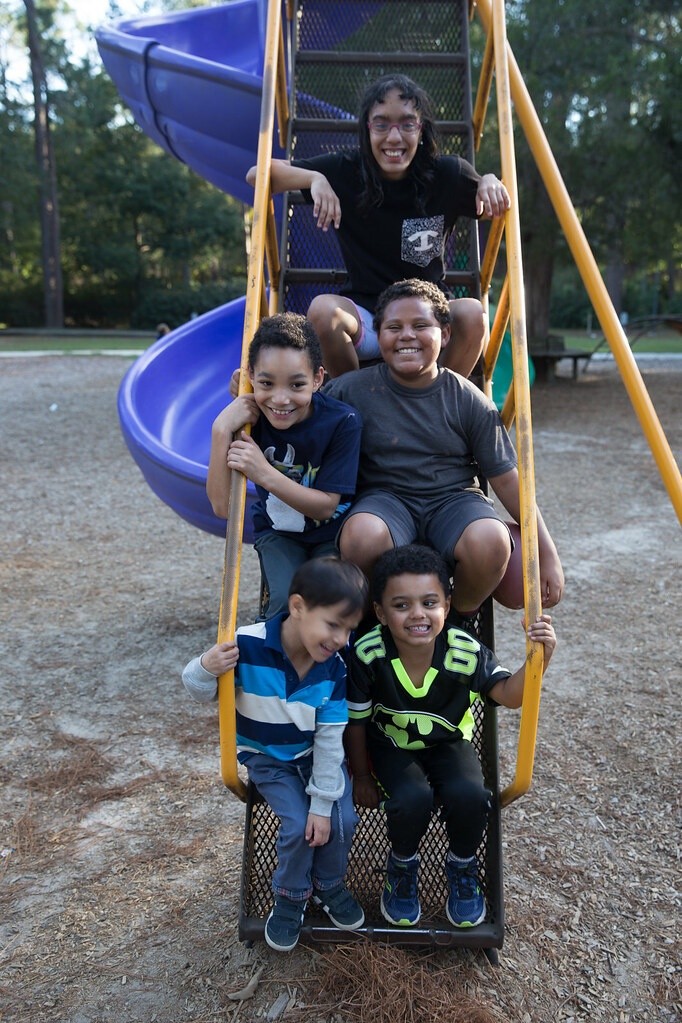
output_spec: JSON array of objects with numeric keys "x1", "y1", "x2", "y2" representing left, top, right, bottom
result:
[
  {"x1": 380, "y1": 849, "x2": 422, "y2": 926},
  {"x1": 445, "y1": 856, "x2": 486, "y2": 928},
  {"x1": 310, "y1": 880, "x2": 365, "y2": 930},
  {"x1": 265, "y1": 893, "x2": 308, "y2": 952}
]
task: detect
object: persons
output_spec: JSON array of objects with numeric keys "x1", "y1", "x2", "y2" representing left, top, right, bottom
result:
[
  {"x1": 205, "y1": 311, "x2": 362, "y2": 620},
  {"x1": 182, "y1": 555, "x2": 365, "y2": 953},
  {"x1": 229, "y1": 279, "x2": 566, "y2": 640},
  {"x1": 247, "y1": 73, "x2": 511, "y2": 380},
  {"x1": 345, "y1": 544, "x2": 556, "y2": 927}
]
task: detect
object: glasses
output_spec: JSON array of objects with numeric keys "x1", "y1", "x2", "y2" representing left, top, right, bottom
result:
[{"x1": 364, "y1": 119, "x2": 424, "y2": 136}]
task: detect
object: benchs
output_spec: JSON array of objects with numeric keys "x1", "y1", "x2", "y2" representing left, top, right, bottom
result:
[{"x1": 531, "y1": 348, "x2": 592, "y2": 383}]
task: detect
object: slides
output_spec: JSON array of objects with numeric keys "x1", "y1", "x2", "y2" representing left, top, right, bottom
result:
[{"x1": 89, "y1": 2, "x2": 385, "y2": 545}]
